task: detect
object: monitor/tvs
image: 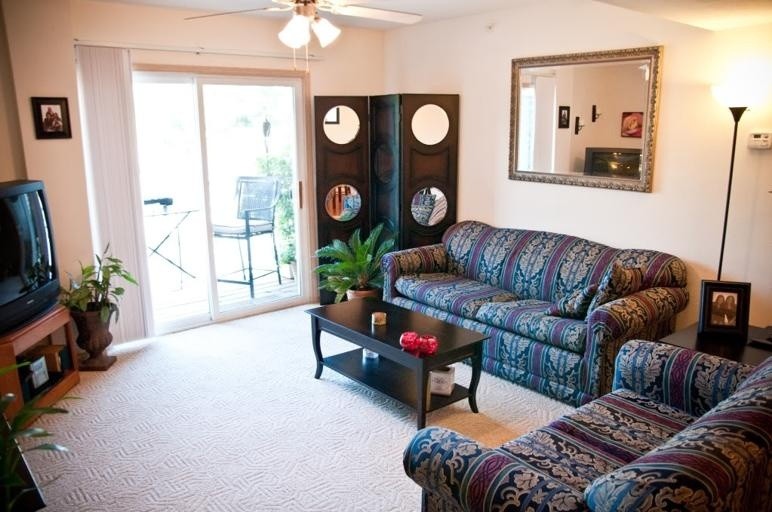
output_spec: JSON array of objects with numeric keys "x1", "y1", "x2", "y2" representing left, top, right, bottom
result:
[
  {"x1": 0, "y1": 179, "x2": 61, "y2": 338},
  {"x1": 584, "y1": 146, "x2": 642, "y2": 180}
]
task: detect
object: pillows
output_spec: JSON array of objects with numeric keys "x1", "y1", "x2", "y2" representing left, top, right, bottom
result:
[
  {"x1": 542, "y1": 283, "x2": 599, "y2": 320},
  {"x1": 582, "y1": 256, "x2": 648, "y2": 324}
]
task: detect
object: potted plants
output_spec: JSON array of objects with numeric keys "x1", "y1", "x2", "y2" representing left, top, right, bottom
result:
[
  {"x1": 308, "y1": 218, "x2": 402, "y2": 304},
  {"x1": 56, "y1": 242, "x2": 141, "y2": 372}
]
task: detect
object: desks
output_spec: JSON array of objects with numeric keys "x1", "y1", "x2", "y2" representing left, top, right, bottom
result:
[
  {"x1": 654, "y1": 318, "x2": 772, "y2": 367},
  {"x1": 144, "y1": 206, "x2": 203, "y2": 279}
]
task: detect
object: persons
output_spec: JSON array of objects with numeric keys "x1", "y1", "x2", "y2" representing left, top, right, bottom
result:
[
  {"x1": 712, "y1": 295, "x2": 725, "y2": 325},
  {"x1": 43, "y1": 107, "x2": 62, "y2": 132},
  {"x1": 725, "y1": 295, "x2": 737, "y2": 326}
]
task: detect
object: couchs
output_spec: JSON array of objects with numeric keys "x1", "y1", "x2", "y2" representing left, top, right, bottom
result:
[
  {"x1": 379, "y1": 220, "x2": 693, "y2": 408},
  {"x1": 402, "y1": 336, "x2": 772, "y2": 512}
]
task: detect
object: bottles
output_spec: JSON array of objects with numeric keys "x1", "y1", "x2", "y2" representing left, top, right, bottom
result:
[
  {"x1": 417, "y1": 334, "x2": 440, "y2": 356},
  {"x1": 400, "y1": 331, "x2": 419, "y2": 351}
]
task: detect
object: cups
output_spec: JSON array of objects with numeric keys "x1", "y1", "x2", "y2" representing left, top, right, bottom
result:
[
  {"x1": 372, "y1": 312, "x2": 387, "y2": 325},
  {"x1": 363, "y1": 347, "x2": 379, "y2": 359}
]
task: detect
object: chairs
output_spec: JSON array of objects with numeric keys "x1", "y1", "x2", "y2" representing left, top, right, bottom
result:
[{"x1": 204, "y1": 172, "x2": 284, "y2": 299}]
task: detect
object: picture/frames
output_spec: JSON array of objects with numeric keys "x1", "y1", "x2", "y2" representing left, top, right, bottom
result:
[
  {"x1": 557, "y1": 104, "x2": 572, "y2": 129},
  {"x1": 696, "y1": 278, "x2": 753, "y2": 345},
  {"x1": 324, "y1": 107, "x2": 341, "y2": 124},
  {"x1": 27, "y1": 93, "x2": 73, "y2": 141}
]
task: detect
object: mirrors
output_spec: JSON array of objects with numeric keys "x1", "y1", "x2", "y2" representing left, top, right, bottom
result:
[
  {"x1": 507, "y1": 43, "x2": 666, "y2": 194},
  {"x1": 322, "y1": 103, "x2": 361, "y2": 146},
  {"x1": 322, "y1": 180, "x2": 365, "y2": 224},
  {"x1": 411, "y1": 103, "x2": 452, "y2": 148},
  {"x1": 406, "y1": 181, "x2": 451, "y2": 227}
]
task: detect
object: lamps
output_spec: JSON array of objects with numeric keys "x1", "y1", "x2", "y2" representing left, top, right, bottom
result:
[
  {"x1": 710, "y1": 55, "x2": 768, "y2": 280},
  {"x1": 278, "y1": 12, "x2": 343, "y2": 69}
]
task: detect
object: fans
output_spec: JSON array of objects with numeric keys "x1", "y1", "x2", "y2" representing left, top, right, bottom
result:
[{"x1": 183, "y1": 0, "x2": 425, "y2": 27}]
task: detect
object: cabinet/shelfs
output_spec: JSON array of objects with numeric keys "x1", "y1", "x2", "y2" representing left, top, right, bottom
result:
[{"x1": 0, "y1": 304, "x2": 83, "y2": 433}]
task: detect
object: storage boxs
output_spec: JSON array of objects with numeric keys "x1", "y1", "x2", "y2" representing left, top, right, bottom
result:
[
  {"x1": 30, "y1": 345, "x2": 67, "y2": 375},
  {"x1": 15, "y1": 353, "x2": 51, "y2": 400},
  {"x1": 429, "y1": 365, "x2": 456, "y2": 397}
]
